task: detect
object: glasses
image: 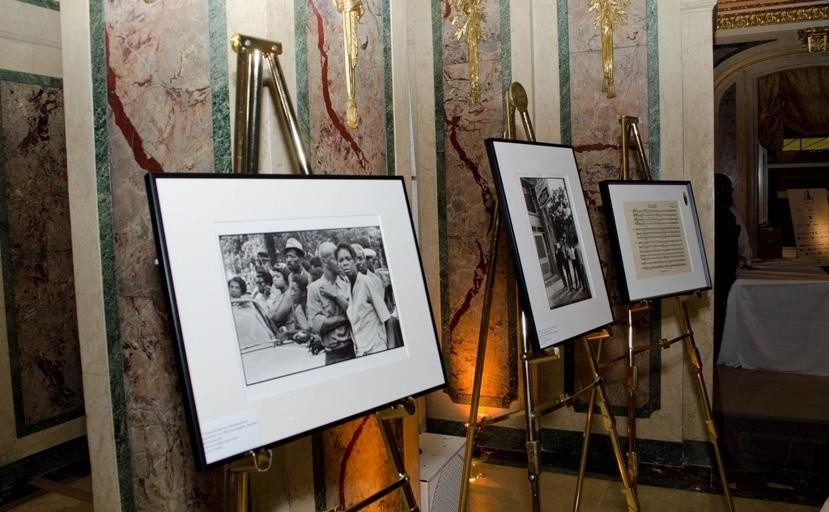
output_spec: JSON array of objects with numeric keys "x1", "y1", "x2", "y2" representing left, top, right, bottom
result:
[{"x1": 256, "y1": 281, "x2": 264, "y2": 285}]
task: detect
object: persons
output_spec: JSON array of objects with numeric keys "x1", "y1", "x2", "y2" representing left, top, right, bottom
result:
[
  {"x1": 228, "y1": 237, "x2": 404, "y2": 366},
  {"x1": 715, "y1": 172, "x2": 738, "y2": 368},
  {"x1": 729, "y1": 187, "x2": 754, "y2": 266},
  {"x1": 554, "y1": 231, "x2": 589, "y2": 294}
]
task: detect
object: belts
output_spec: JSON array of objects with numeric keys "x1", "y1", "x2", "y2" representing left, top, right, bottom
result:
[{"x1": 324, "y1": 340, "x2": 351, "y2": 352}]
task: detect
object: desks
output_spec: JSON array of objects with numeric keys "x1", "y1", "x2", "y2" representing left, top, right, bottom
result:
[{"x1": 733, "y1": 254, "x2": 828, "y2": 377}]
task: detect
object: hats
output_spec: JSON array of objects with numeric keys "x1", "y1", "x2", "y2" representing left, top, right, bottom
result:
[
  {"x1": 364, "y1": 248, "x2": 377, "y2": 259},
  {"x1": 283, "y1": 238, "x2": 305, "y2": 257}
]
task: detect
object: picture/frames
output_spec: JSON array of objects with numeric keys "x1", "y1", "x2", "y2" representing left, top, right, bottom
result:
[
  {"x1": 145, "y1": 171, "x2": 447, "y2": 472},
  {"x1": 600, "y1": 178, "x2": 712, "y2": 304},
  {"x1": 485, "y1": 135, "x2": 615, "y2": 351}
]
